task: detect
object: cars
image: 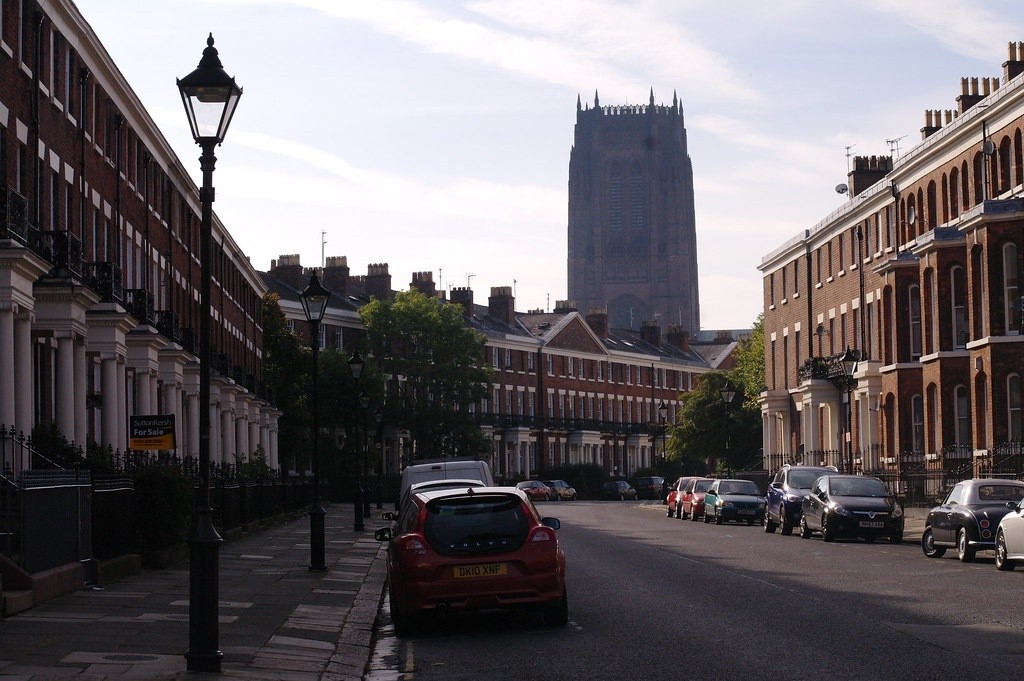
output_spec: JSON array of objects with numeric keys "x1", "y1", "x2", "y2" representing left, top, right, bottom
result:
[
  {"x1": 921, "y1": 479, "x2": 1024, "y2": 561},
  {"x1": 542, "y1": 480, "x2": 577, "y2": 501},
  {"x1": 375, "y1": 479, "x2": 566, "y2": 636},
  {"x1": 635, "y1": 476, "x2": 672, "y2": 500},
  {"x1": 800, "y1": 475, "x2": 905, "y2": 545},
  {"x1": 516, "y1": 481, "x2": 550, "y2": 501},
  {"x1": 764, "y1": 464, "x2": 839, "y2": 535},
  {"x1": 602, "y1": 481, "x2": 639, "y2": 501},
  {"x1": 665, "y1": 476, "x2": 765, "y2": 525},
  {"x1": 993, "y1": 496, "x2": 1024, "y2": 571}
]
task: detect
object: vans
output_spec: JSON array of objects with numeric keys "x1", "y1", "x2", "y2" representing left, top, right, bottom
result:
[{"x1": 395, "y1": 460, "x2": 494, "y2": 511}]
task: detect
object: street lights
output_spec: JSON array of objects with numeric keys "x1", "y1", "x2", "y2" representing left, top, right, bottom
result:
[
  {"x1": 658, "y1": 399, "x2": 670, "y2": 504},
  {"x1": 175, "y1": 30, "x2": 244, "y2": 673},
  {"x1": 719, "y1": 381, "x2": 738, "y2": 477},
  {"x1": 346, "y1": 350, "x2": 370, "y2": 532},
  {"x1": 297, "y1": 270, "x2": 332, "y2": 572},
  {"x1": 837, "y1": 345, "x2": 859, "y2": 474}
]
